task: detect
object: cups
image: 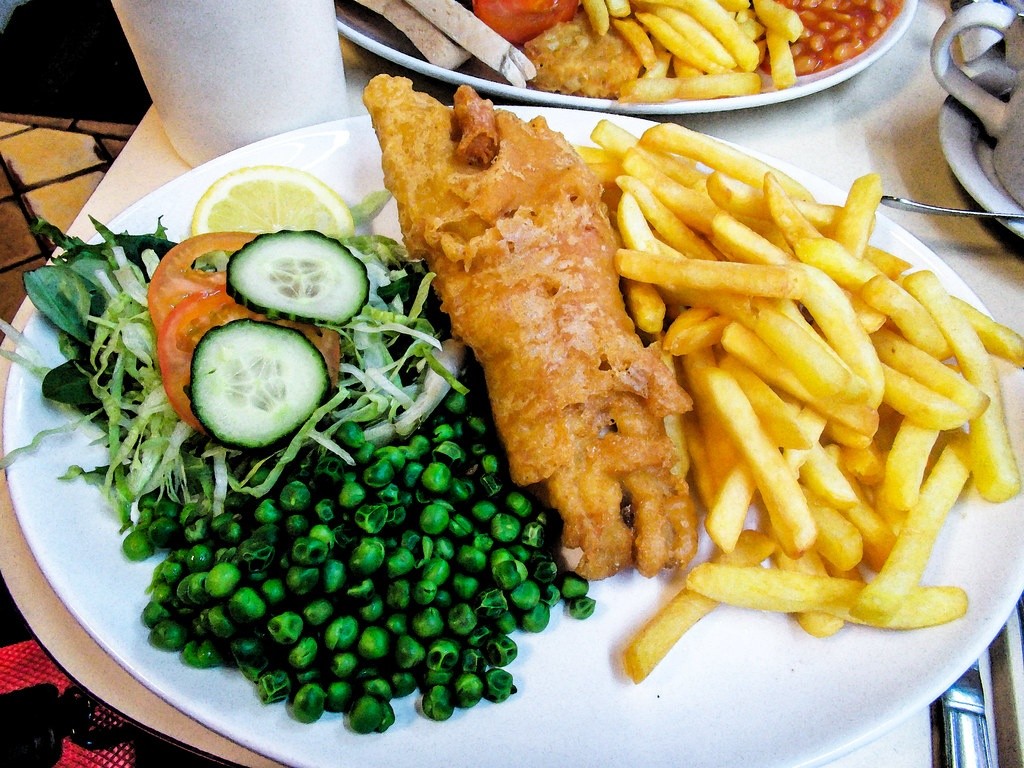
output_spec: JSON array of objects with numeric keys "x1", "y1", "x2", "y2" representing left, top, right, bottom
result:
[
  {"x1": 930, "y1": 0, "x2": 1024, "y2": 208},
  {"x1": 110, "y1": 0, "x2": 348, "y2": 169}
]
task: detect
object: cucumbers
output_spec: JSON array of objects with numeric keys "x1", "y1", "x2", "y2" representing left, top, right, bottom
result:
[
  {"x1": 224, "y1": 230, "x2": 371, "y2": 324},
  {"x1": 188, "y1": 317, "x2": 331, "y2": 450}
]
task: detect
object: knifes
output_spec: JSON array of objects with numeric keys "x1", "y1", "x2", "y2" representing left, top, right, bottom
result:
[{"x1": 943, "y1": 659, "x2": 991, "y2": 768}]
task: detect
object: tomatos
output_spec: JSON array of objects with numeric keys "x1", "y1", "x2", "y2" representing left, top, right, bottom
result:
[
  {"x1": 156, "y1": 285, "x2": 340, "y2": 435},
  {"x1": 146, "y1": 231, "x2": 261, "y2": 328},
  {"x1": 468, "y1": 0, "x2": 581, "y2": 45}
]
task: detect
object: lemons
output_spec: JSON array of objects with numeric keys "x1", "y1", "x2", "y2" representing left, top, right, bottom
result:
[{"x1": 190, "y1": 163, "x2": 355, "y2": 238}]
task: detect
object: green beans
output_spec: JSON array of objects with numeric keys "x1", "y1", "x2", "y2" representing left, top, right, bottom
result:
[{"x1": 122, "y1": 390, "x2": 596, "y2": 733}]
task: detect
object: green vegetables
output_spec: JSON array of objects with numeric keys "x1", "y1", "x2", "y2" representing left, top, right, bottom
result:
[{"x1": 0, "y1": 211, "x2": 470, "y2": 524}]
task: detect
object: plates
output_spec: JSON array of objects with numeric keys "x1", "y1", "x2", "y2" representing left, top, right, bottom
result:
[
  {"x1": 938, "y1": 68, "x2": 1024, "y2": 240},
  {"x1": 0, "y1": 104, "x2": 1024, "y2": 768},
  {"x1": 333, "y1": 0, "x2": 917, "y2": 118}
]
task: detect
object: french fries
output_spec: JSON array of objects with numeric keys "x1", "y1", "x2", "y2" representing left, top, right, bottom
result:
[
  {"x1": 559, "y1": 118, "x2": 1024, "y2": 685},
  {"x1": 583, "y1": 0, "x2": 805, "y2": 103}
]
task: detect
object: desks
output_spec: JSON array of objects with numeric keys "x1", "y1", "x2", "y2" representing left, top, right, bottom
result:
[{"x1": 0, "y1": 0, "x2": 1024, "y2": 768}]
